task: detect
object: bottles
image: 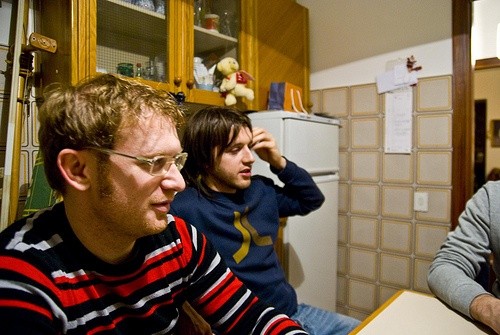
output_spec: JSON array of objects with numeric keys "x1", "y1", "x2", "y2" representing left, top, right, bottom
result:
[
  {"x1": 131, "y1": 0, "x2": 166, "y2": 17},
  {"x1": 117, "y1": 63, "x2": 134, "y2": 77},
  {"x1": 135, "y1": 63, "x2": 156, "y2": 81},
  {"x1": 218, "y1": 9, "x2": 236, "y2": 38}
]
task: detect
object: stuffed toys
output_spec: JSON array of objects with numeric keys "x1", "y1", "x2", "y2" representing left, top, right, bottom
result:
[{"x1": 217, "y1": 57, "x2": 255, "y2": 105}]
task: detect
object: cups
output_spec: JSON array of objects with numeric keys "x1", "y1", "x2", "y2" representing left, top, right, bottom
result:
[
  {"x1": 196, "y1": 84, "x2": 214, "y2": 91},
  {"x1": 204, "y1": 13, "x2": 220, "y2": 34}
]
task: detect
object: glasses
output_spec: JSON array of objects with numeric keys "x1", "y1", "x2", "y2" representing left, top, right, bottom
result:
[{"x1": 80, "y1": 146, "x2": 188, "y2": 177}]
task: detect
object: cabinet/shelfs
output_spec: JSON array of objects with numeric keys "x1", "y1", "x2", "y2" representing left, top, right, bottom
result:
[{"x1": 34, "y1": 0, "x2": 259, "y2": 111}]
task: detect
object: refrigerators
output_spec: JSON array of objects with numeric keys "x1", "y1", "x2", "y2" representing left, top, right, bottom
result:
[{"x1": 242, "y1": 109, "x2": 342, "y2": 312}]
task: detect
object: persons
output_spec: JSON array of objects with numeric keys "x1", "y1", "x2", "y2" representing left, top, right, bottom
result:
[
  {"x1": 167, "y1": 106, "x2": 362, "y2": 335},
  {"x1": 427, "y1": 181, "x2": 500, "y2": 335},
  {"x1": 0, "y1": 72, "x2": 311, "y2": 335}
]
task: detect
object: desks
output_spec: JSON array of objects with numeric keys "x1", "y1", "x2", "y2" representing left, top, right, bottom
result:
[{"x1": 345, "y1": 288, "x2": 498, "y2": 335}]
